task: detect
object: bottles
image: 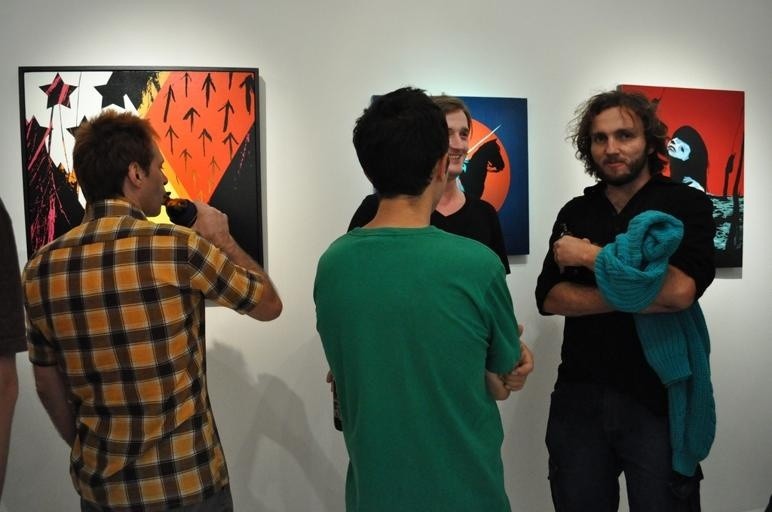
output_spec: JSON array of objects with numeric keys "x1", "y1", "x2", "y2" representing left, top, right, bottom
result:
[
  {"x1": 161, "y1": 195, "x2": 197, "y2": 228},
  {"x1": 561, "y1": 222, "x2": 581, "y2": 276}
]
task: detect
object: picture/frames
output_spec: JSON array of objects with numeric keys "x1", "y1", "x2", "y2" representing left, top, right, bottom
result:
[{"x1": 18, "y1": 66, "x2": 263, "y2": 299}]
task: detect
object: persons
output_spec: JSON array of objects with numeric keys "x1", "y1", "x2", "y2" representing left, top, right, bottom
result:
[
  {"x1": 2, "y1": 199, "x2": 30, "y2": 504},
  {"x1": 23, "y1": 107, "x2": 285, "y2": 512},
  {"x1": 311, "y1": 85, "x2": 534, "y2": 512},
  {"x1": 536, "y1": 90, "x2": 719, "y2": 510},
  {"x1": 346, "y1": 94, "x2": 512, "y2": 277}
]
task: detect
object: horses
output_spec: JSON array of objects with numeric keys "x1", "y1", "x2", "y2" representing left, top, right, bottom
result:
[{"x1": 459, "y1": 138, "x2": 505, "y2": 199}]
